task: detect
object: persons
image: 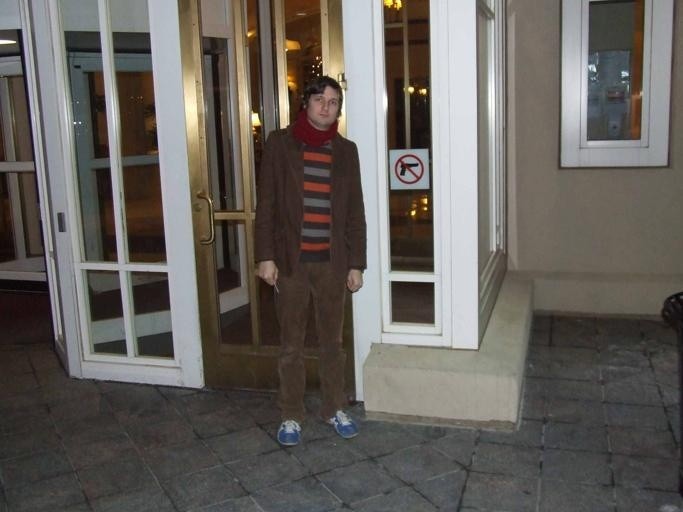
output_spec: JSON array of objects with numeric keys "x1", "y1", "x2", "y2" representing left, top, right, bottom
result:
[{"x1": 252, "y1": 76, "x2": 367, "y2": 447}]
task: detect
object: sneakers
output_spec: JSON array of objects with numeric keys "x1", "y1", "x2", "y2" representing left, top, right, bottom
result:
[
  {"x1": 277, "y1": 418, "x2": 302, "y2": 446},
  {"x1": 325, "y1": 409, "x2": 359, "y2": 439}
]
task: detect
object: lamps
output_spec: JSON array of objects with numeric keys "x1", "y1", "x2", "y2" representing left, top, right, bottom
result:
[{"x1": 252, "y1": 113, "x2": 261, "y2": 146}]
